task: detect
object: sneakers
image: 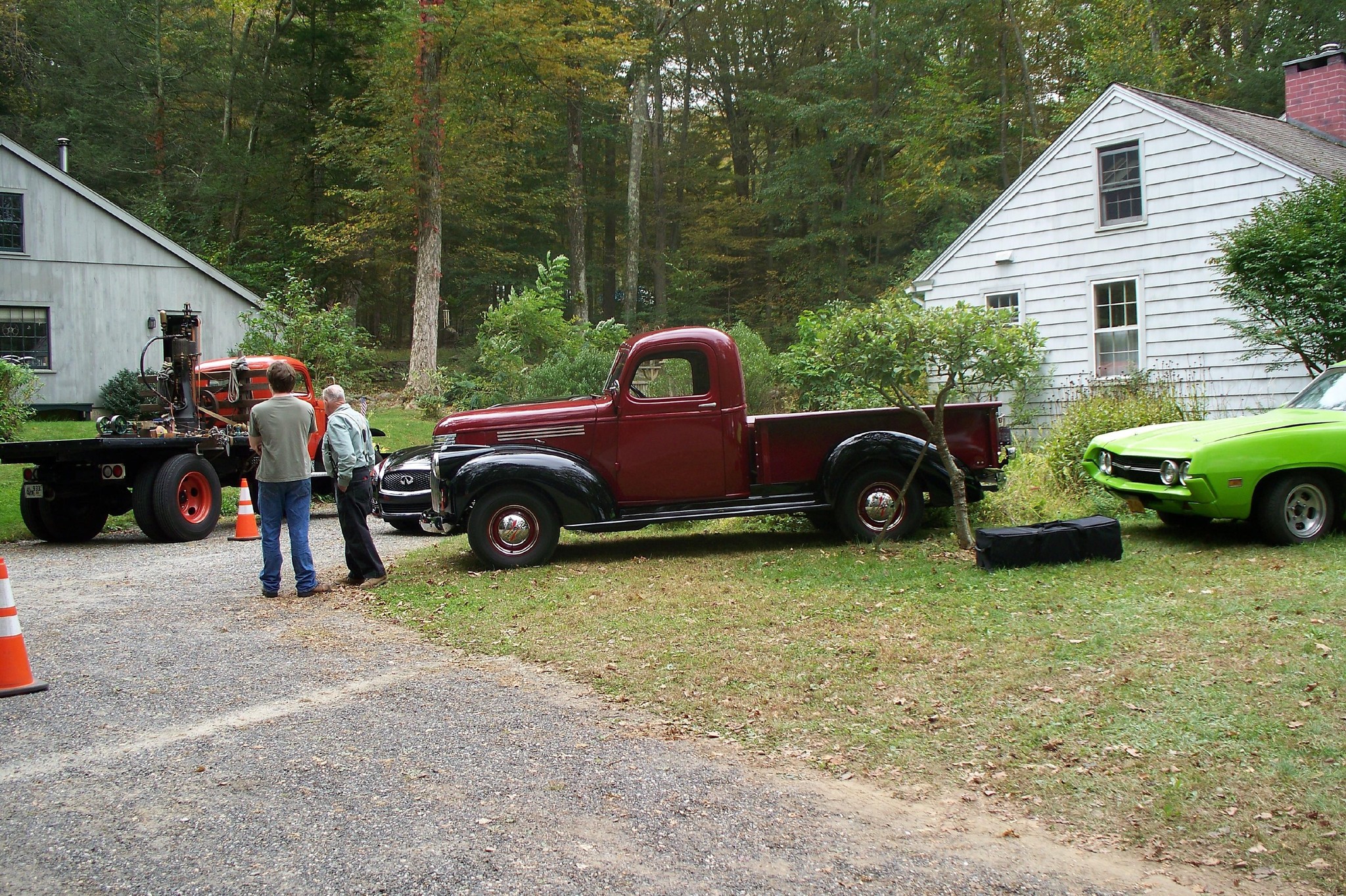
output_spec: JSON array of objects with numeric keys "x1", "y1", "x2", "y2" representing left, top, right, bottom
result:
[
  {"x1": 359, "y1": 575, "x2": 388, "y2": 589},
  {"x1": 262, "y1": 588, "x2": 278, "y2": 597},
  {"x1": 334, "y1": 575, "x2": 360, "y2": 585},
  {"x1": 298, "y1": 581, "x2": 329, "y2": 597}
]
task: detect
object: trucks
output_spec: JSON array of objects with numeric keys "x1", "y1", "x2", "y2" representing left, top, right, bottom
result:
[{"x1": 0, "y1": 301, "x2": 387, "y2": 546}]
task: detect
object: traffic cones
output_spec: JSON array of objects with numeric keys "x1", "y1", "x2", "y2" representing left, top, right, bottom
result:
[
  {"x1": 226, "y1": 477, "x2": 262, "y2": 542},
  {"x1": 0, "y1": 556, "x2": 50, "y2": 697}
]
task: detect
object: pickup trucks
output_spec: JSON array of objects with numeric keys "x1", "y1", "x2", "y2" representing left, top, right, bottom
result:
[{"x1": 418, "y1": 322, "x2": 1020, "y2": 572}]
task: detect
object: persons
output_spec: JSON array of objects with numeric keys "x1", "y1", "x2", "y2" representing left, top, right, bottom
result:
[
  {"x1": 322, "y1": 384, "x2": 388, "y2": 588},
  {"x1": 249, "y1": 362, "x2": 329, "y2": 597}
]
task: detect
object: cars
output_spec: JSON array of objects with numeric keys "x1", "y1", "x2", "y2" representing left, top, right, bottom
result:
[
  {"x1": 1078, "y1": 357, "x2": 1346, "y2": 548},
  {"x1": 366, "y1": 393, "x2": 607, "y2": 537}
]
